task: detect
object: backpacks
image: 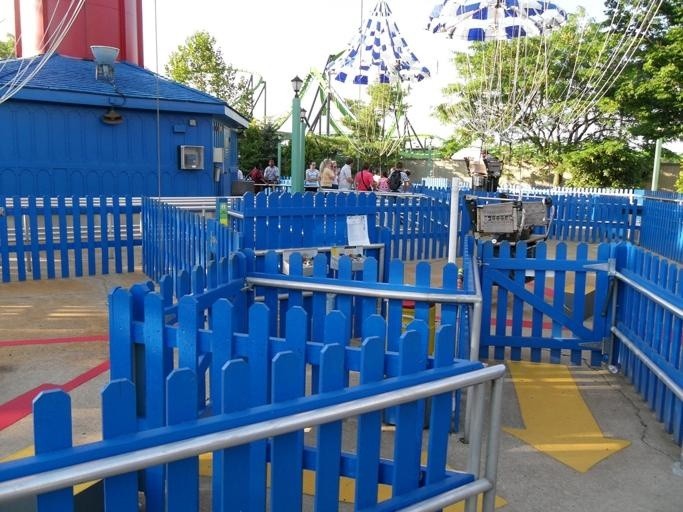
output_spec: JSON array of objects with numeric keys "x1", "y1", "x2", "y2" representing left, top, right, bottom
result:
[{"x1": 389, "y1": 170, "x2": 402, "y2": 190}]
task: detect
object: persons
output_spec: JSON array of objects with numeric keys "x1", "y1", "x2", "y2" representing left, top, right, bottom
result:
[
  {"x1": 304, "y1": 157, "x2": 411, "y2": 193},
  {"x1": 238, "y1": 168, "x2": 243, "y2": 180},
  {"x1": 264, "y1": 158, "x2": 281, "y2": 184},
  {"x1": 243, "y1": 161, "x2": 274, "y2": 194}
]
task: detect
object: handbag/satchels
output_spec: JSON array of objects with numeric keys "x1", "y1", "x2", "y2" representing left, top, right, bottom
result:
[{"x1": 367, "y1": 186, "x2": 372, "y2": 191}]
